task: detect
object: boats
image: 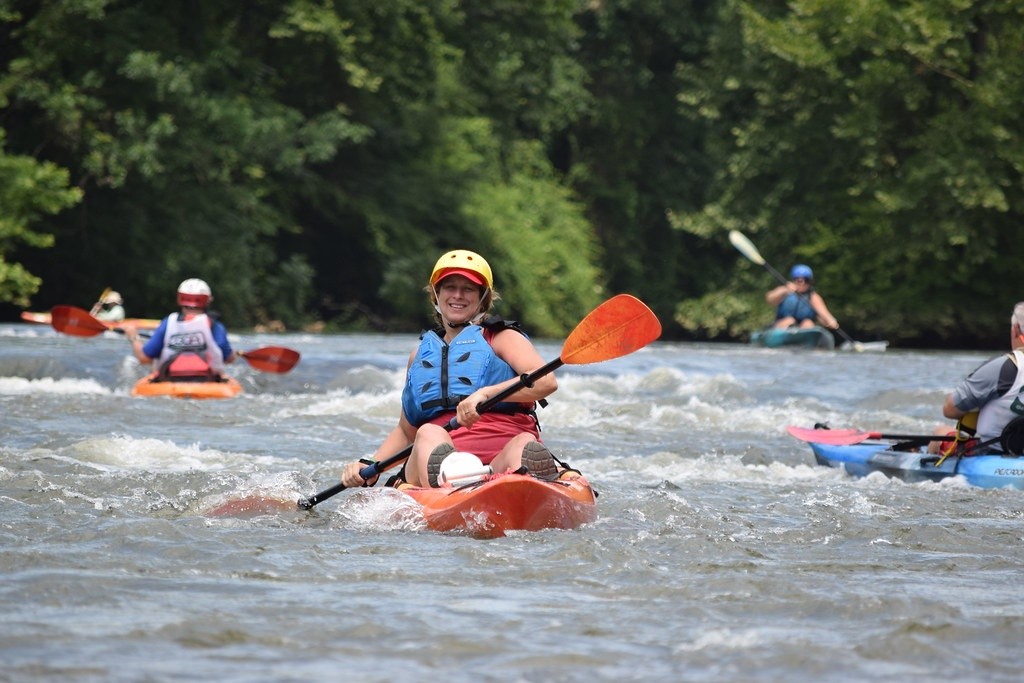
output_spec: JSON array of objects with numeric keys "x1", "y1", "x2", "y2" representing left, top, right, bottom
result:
[
  {"x1": 134, "y1": 370, "x2": 242, "y2": 399},
  {"x1": 809, "y1": 420, "x2": 1024, "y2": 492},
  {"x1": 748, "y1": 325, "x2": 837, "y2": 351},
  {"x1": 397, "y1": 474, "x2": 598, "y2": 541},
  {"x1": 21, "y1": 311, "x2": 163, "y2": 329}
]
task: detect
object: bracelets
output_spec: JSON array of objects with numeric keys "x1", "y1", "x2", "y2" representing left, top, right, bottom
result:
[{"x1": 129, "y1": 336, "x2": 140, "y2": 342}]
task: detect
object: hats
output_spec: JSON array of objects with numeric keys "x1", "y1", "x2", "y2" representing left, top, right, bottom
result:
[{"x1": 432, "y1": 268, "x2": 488, "y2": 290}]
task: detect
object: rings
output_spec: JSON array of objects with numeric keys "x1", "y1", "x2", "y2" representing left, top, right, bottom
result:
[{"x1": 465, "y1": 411, "x2": 469, "y2": 414}]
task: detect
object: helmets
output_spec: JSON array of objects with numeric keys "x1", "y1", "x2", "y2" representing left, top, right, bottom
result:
[
  {"x1": 176, "y1": 278, "x2": 212, "y2": 308},
  {"x1": 100, "y1": 291, "x2": 124, "y2": 306},
  {"x1": 428, "y1": 249, "x2": 493, "y2": 306},
  {"x1": 790, "y1": 264, "x2": 813, "y2": 280}
]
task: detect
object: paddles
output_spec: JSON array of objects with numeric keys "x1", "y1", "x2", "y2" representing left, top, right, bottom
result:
[
  {"x1": 50, "y1": 305, "x2": 300, "y2": 376},
  {"x1": 729, "y1": 229, "x2": 883, "y2": 351},
  {"x1": 91, "y1": 286, "x2": 113, "y2": 316},
  {"x1": 781, "y1": 425, "x2": 965, "y2": 448},
  {"x1": 212, "y1": 293, "x2": 664, "y2": 516}
]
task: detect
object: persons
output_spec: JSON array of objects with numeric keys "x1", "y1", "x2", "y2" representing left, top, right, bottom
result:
[
  {"x1": 89, "y1": 291, "x2": 124, "y2": 332},
  {"x1": 765, "y1": 264, "x2": 839, "y2": 331},
  {"x1": 120, "y1": 278, "x2": 239, "y2": 380},
  {"x1": 341, "y1": 249, "x2": 559, "y2": 488},
  {"x1": 928, "y1": 302, "x2": 1024, "y2": 455}
]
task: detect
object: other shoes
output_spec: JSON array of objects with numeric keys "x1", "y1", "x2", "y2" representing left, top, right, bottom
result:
[
  {"x1": 426, "y1": 442, "x2": 458, "y2": 488},
  {"x1": 521, "y1": 441, "x2": 559, "y2": 480}
]
